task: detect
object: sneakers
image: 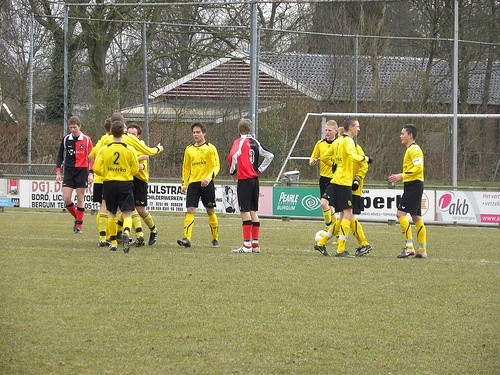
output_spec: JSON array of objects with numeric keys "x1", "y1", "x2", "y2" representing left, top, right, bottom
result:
[
  {"x1": 332, "y1": 237, "x2": 339, "y2": 245},
  {"x1": 232, "y1": 245, "x2": 252, "y2": 253},
  {"x1": 353, "y1": 244, "x2": 372, "y2": 257},
  {"x1": 149, "y1": 228, "x2": 158, "y2": 245},
  {"x1": 122, "y1": 233, "x2": 130, "y2": 253},
  {"x1": 314, "y1": 243, "x2": 331, "y2": 256},
  {"x1": 335, "y1": 250, "x2": 355, "y2": 258},
  {"x1": 107, "y1": 239, "x2": 112, "y2": 244},
  {"x1": 409, "y1": 252, "x2": 426, "y2": 258},
  {"x1": 252, "y1": 246, "x2": 260, "y2": 253},
  {"x1": 109, "y1": 244, "x2": 116, "y2": 250},
  {"x1": 97, "y1": 241, "x2": 105, "y2": 247},
  {"x1": 325, "y1": 225, "x2": 331, "y2": 231},
  {"x1": 397, "y1": 247, "x2": 415, "y2": 258},
  {"x1": 117, "y1": 232, "x2": 122, "y2": 242},
  {"x1": 177, "y1": 238, "x2": 191, "y2": 247},
  {"x1": 212, "y1": 239, "x2": 221, "y2": 247},
  {"x1": 131, "y1": 238, "x2": 145, "y2": 246}
]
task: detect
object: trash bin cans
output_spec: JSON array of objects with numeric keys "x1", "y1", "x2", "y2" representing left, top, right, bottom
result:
[{"x1": 283, "y1": 170, "x2": 300, "y2": 188}]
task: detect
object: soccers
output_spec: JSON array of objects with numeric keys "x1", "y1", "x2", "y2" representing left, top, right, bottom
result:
[{"x1": 315, "y1": 230, "x2": 327, "y2": 242}]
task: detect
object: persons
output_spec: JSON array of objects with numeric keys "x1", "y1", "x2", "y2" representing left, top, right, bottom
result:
[
  {"x1": 308, "y1": 120, "x2": 338, "y2": 233},
  {"x1": 228, "y1": 119, "x2": 274, "y2": 252},
  {"x1": 177, "y1": 122, "x2": 220, "y2": 247},
  {"x1": 389, "y1": 124, "x2": 427, "y2": 258},
  {"x1": 89, "y1": 113, "x2": 164, "y2": 253},
  {"x1": 56, "y1": 116, "x2": 94, "y2": 233},
  {"x1": 337, "y1": 127, "x2": 372, "y2": 257},
  {"x1": 313, "y1": 118, "x2": 373, "y2": 258}
]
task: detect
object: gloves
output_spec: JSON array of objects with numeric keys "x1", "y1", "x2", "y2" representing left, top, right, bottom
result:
[
  {"x1": 352, "y1": 180, "x2": 358, "y2": 191},
  {"x1": 368, "y1": 157, "x2": 372, "y2": 166}
]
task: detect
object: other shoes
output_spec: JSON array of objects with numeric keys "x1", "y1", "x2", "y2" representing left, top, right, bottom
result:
[
  {"x1": 73, "y1": 222, "x2": 76, "y2": 231},
  {"x1": 75, "y1": 229, "x2": 82, "y2": 234}
]
